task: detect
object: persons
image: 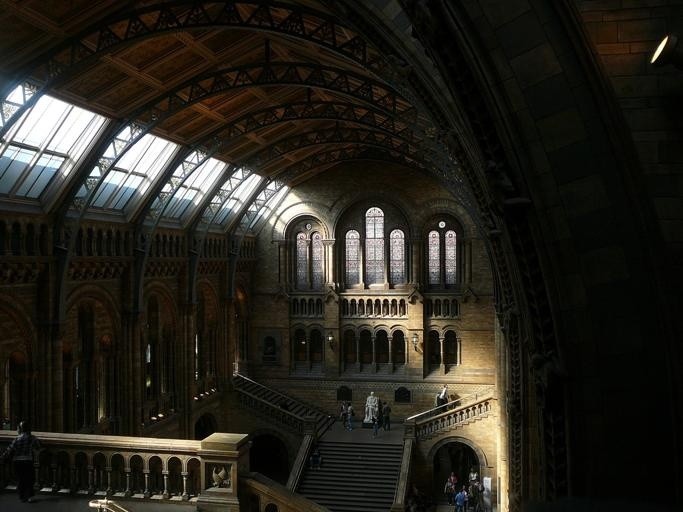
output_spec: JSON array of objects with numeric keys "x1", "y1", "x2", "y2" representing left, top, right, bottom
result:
[
  {"x1": 382, "y1": 402, "x2": 391, "y2": 432},
  {"x1": 372, "y1": 412, "x2": 379, "y2": 437},
  {"x1": 365, "y1": 392, "x2": 378, "y2": 419},
  {"x1": 444, "y1": 468, "x2": 483, "y2": 512},
  {"x1": 0, "y1": 421, "x2": 42, "y2": 503},
  {"x1": 435, "y1": 384, "x2": 448, "y2": 407},
  {"x1": 341, "y1": 401, "x2": 352, "y2": 432}
]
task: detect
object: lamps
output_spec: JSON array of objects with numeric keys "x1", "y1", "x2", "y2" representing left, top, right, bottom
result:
[
  {"x1": 329, "y1": 332, "x2": 336, "y2": 351},
  {"x1": 413, "y1": 333, "x2": 419, "y2": 352}
]
